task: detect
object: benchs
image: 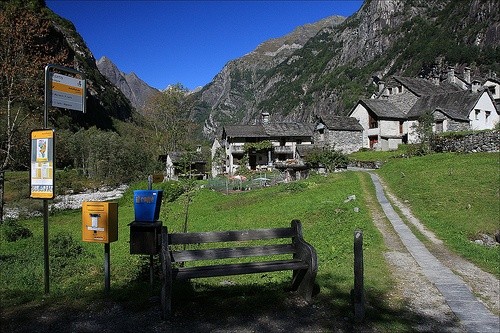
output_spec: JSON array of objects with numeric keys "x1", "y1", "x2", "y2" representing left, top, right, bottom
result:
[{"x1": 152, "y1": 219, "x2": 319, "y2": 318}]
